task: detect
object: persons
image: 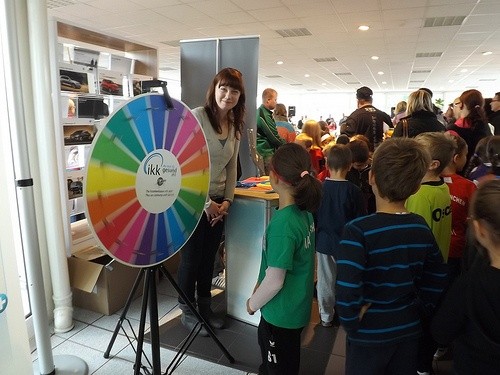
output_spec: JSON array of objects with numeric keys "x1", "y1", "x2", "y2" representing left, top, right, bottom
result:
[
  {"x1": 210, "y1": 153, "x2": 242, "y2": 290},
  {"x1": 177, "y1": 68, "x2": 247, "y2": 338},
  {"x1": 246, "y1": 86, "x2": 500, "y2": 375}
]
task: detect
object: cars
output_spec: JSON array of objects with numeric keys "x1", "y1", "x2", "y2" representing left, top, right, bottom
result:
[
  {"x1": 65, "y1": 129, "x2": 92, "y2": 143},
  {"x1": 69, "y1": 180, "x2": 84, "y2": 195},
  {"x1": 60, "y1": 74, "x2": 81, "y2": 89}
]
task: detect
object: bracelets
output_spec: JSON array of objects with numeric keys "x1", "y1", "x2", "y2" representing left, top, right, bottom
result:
[{"x1": 222, "y1": 198, "x2": 232, "y2": 206}]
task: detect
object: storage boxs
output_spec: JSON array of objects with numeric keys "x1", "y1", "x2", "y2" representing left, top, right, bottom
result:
[{"x1": 69, "y1": 244, "x2": 161, "y2": 317}]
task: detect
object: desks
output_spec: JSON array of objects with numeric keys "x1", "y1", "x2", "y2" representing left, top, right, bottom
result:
[{"x1": 225, "y1": 176, "x2": 280, "y2": 327}]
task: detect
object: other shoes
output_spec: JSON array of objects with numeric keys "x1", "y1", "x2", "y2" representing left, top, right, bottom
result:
[
  {"x1": 321, "y1": 320, "x2": 332, "y2": 327},
  {"x1": 417, "y1": 365, "x2": 432, "y2": 375},
  {"x1": 434, "y1": 347, "x2": 450, "y2": 359}
]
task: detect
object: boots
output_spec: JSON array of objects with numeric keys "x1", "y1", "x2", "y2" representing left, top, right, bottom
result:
[
  {"x1": 180, "y1": 303, "x2": 209, "y2": 336},
  {"x1": 196, "y1": 297, "x2": 224, "y2": 329}
]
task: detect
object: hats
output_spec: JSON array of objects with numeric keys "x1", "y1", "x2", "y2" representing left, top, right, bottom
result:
[{"x1": 357, "y1": 87, "x2": 373, "y2": 98}]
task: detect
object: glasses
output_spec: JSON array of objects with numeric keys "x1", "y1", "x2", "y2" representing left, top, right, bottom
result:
[
  {"x1": 492, "y1": 99, "x2": 500, "y2": 102},
  {"x1": 454, "y1": 102, "x2": 461, "y2": 107}
]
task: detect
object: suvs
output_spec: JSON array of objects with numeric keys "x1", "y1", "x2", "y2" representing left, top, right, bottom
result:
[{"x1": 100, "y1": 78, "x2": 120, "y2": 92}]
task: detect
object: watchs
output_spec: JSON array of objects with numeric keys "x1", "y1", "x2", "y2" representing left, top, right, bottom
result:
[{"x1": 204, "y1": 199, "x2": 212, "y2": 209}]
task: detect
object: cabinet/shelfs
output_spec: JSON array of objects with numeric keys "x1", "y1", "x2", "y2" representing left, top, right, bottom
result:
[{"x1": 47, "y1": 15, "x2": 160, "y2": 259}]
